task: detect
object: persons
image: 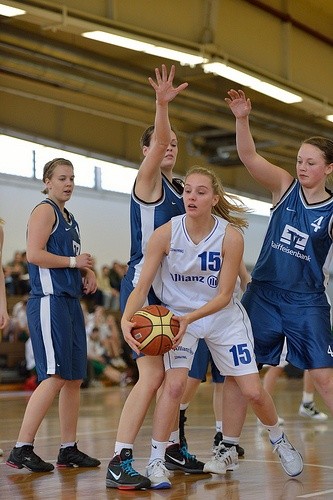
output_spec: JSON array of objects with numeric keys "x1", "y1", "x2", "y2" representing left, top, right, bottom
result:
[
  {"x1": 81, "y1": 261, "x2": 139, "y2": 387},
  {"x1": 258, "y1": 336, "x2": 328, "y2": 426},
  {"x1": 105, "y1": 64, "x2": 210, "y2": 491},
  {"x1": 0, "y1": 219, "x2": 40, "y2": 390},
  {"x1": 6, "y1": 158, "x2": 101, "y2": 473},
  {"x1": 120, "y1": 166, "x2": 304, "y2": 490},
  {"x1": 179, "y1": 255, "x2": 251, "y2": 456},
  {"x1": 201, "y1": 89, "x2": 333, "y2": 475}
]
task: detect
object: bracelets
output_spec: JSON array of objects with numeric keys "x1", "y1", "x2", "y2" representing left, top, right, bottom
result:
[{"x1": 69, "y1": 257, "x2": 76, "y2": 268}]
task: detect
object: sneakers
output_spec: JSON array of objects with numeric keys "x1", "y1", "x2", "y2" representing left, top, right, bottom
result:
[
  {"x1": 270, "y1": 432, "x2": 304, "y2": 477},
  {"x1": 214, "y1": 432, "x2": 244, "y2": 456},
  {"x1": 203, "y1": 444, "x2": 239, "y2": 474},
  {"x1": 146, "y1": 459, "x2": 172, "y2": 489},
  {"x1": 105, "y1": 448, "x2": 151, "y2": 490},
  {"x1": 6, "y1": 445, "x2": 55, "y2": 473},
  {"x1": 56, "y1": 441, "x2": 101, "y2": 468},
  {"x1": 164, "y1": 444, "x2": 209, "y2": 474},
  {"x1": 279, "y1": 418, "x2": 285, "y2": 425},
  {"x1": 178, "y1": 410, "x2": 188, "y2": 450},
  {"x1": 299, "y1": 404, "x2": 328, "y2": 421}
]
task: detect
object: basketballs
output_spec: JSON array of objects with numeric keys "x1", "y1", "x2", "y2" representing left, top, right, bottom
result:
[{"x1": 130, "y1": 305, "x2": 180, "y2": 357}]
